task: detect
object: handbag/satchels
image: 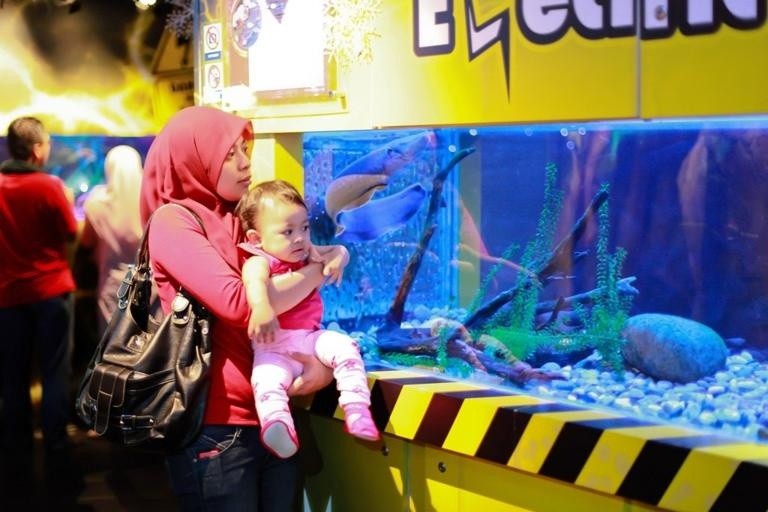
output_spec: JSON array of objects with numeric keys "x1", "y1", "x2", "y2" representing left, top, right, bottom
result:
[{"x1": 73, "y1": 203, "x2": 213, "y2": 455}]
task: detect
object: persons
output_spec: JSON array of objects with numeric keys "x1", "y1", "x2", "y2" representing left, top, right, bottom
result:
[
  {"x1": 79, "y1": 145, "x2": 146, "y2": 341},
  {"x1": 239, "y1": 181, "x2": 380, "y2": 459},
  {"x1": 139, "y1": 106, "x2": 335, "y2": 512},
  {"x1": 0, "y1": 117, "x2": 78, "y2": 462}
]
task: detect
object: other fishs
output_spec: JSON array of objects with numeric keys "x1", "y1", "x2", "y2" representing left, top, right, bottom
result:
[
  {"x1": 446, "y1": 339, "x2": 488, "y2": 372},
  {"x1": 333, "y1": 131, "x2": 433, "y2": 179},
  {"x1": 338, "y1": 181, "x2": 428, "y2": 242},
  {"x1": 323, "y1": 172, "x2": 389, "y2": 239},
  {"x1": 420, "y1": 316, "x2": 473, "y2": 343}
]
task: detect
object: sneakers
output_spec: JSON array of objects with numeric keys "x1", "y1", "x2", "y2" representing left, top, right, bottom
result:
[
  {"x1": 344, "y1": 407, "x2": 380, "y2": 443},
  {"x1": 259, "y1": 419, "x2": 300, "y2": 459}
]
